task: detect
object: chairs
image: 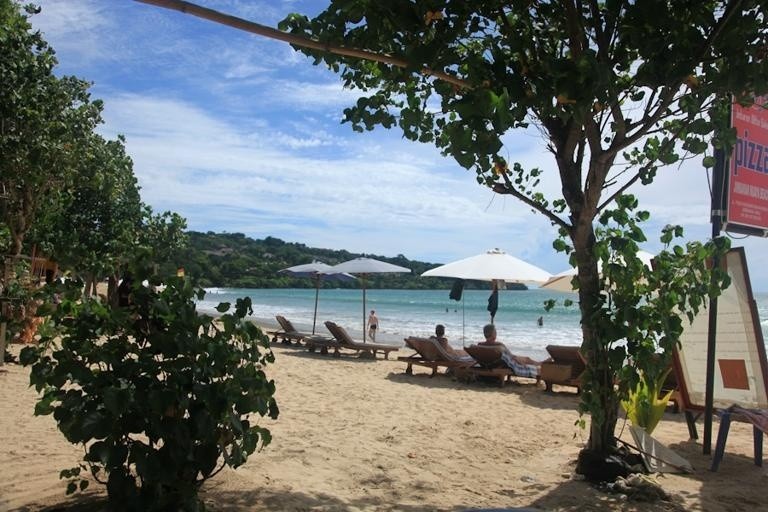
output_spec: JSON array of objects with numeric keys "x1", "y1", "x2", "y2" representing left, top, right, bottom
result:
[
  {"x1": 397, "y1": 336, "x2": 684, "y2": 414},
  {"x1": 266, "y1": 314, "x2": 398, "y2": 360}
]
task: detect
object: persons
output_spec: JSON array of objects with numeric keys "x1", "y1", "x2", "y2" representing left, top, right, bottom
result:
[
  {"x1": 428, "y1": 323, "x2": 553, "y2": 369},
  {"x1": 537, "y1": 316, "x2": 543, "y2": 328},
  {"x1": 366, "y1": 309, "x2": 378, "y2": 342}
]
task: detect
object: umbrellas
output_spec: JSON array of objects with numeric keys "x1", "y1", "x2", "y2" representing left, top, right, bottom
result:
[
  {"x1": 277, "y1": 261, "x2": 357, "y2": 335},
  {"x1": 420, "y1": 248, "x2": 553, "y2": 349},
  {"x1": 315, "y1": 257, "x2": 411, "y2": 342},
  {"x1": 537, "y1": 250, "x2": 727, "y2": 365}
]
